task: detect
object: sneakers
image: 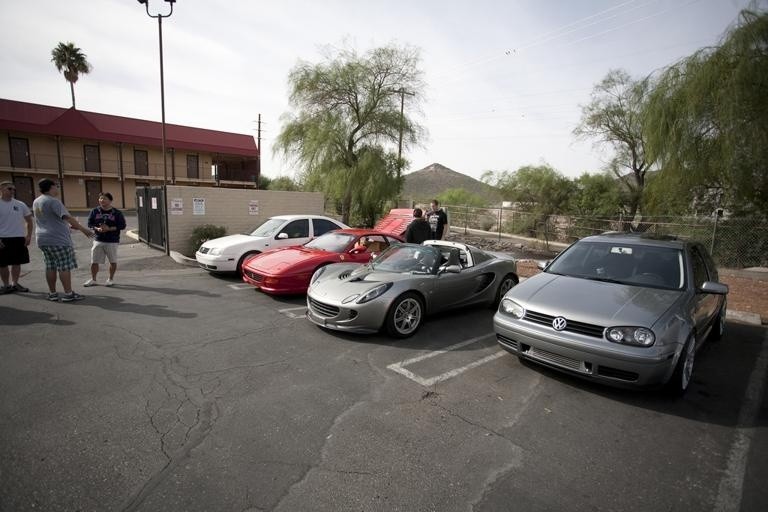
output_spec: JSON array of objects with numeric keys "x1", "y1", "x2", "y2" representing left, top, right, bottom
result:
[
  {"x1": 104, "y1": 280, "x2": 112, "y2": 287},
  {"x1": 12, "y1": 283, "x2": 28, "y2": 292},
  {"x1": 45, "y1": 293, "x2": 58, "y2": 301},
  {"x1": 0, "y1": 285, "x2": 12, "y2": 295},
  {"x1": 83, "y1": 279, "x2": 97, "y2": 287},
  {"x1": 60, "y1": 290, "x2": 86, "y2": 302}
]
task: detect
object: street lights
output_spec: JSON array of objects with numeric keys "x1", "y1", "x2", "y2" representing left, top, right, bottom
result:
[{"x1": 136, "y1": 0, "x2": 177, "y2": 186}]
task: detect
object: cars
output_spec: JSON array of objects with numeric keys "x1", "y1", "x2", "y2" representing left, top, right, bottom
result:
[
  {"x1": 193, "y1": 213, "x2": 353, "y2": 275},
  {"x1": 490, "y1": 228, "x2": 731, "y2": 397}
]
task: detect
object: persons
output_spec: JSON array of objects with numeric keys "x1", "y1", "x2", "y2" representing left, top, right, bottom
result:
[
  {"x1": 0, "y1": 182, "x2": 33, "y2": 295},
  {"x1": 83, "y1": 193, "x2": 126, "y2": 287},
  {"x1": 33, "y1": 179, "x2": 96, "y2": 302},
  {"x1": 407, "y1": 209, "x2": 429, "y2": 244},
  {"x1": 427, "y1": 200, "x2": 447, "y2": 240}
]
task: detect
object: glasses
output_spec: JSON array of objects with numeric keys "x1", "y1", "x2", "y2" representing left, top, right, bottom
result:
[{"x1": 6, "y1": 187, "x2": 16, "y2": 192}]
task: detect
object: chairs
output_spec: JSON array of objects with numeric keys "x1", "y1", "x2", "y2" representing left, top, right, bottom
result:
[{"x1": 441, "y1": 247, "x2": 464, "y2": 270}]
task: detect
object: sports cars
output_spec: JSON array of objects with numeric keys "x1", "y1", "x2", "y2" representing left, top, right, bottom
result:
[
  {"x1": 241, "y1": 209, "x2": 423, "y2": 294},
  {"x1": 304, "y1": 239, "x2": 520, "y2": 338}
]
